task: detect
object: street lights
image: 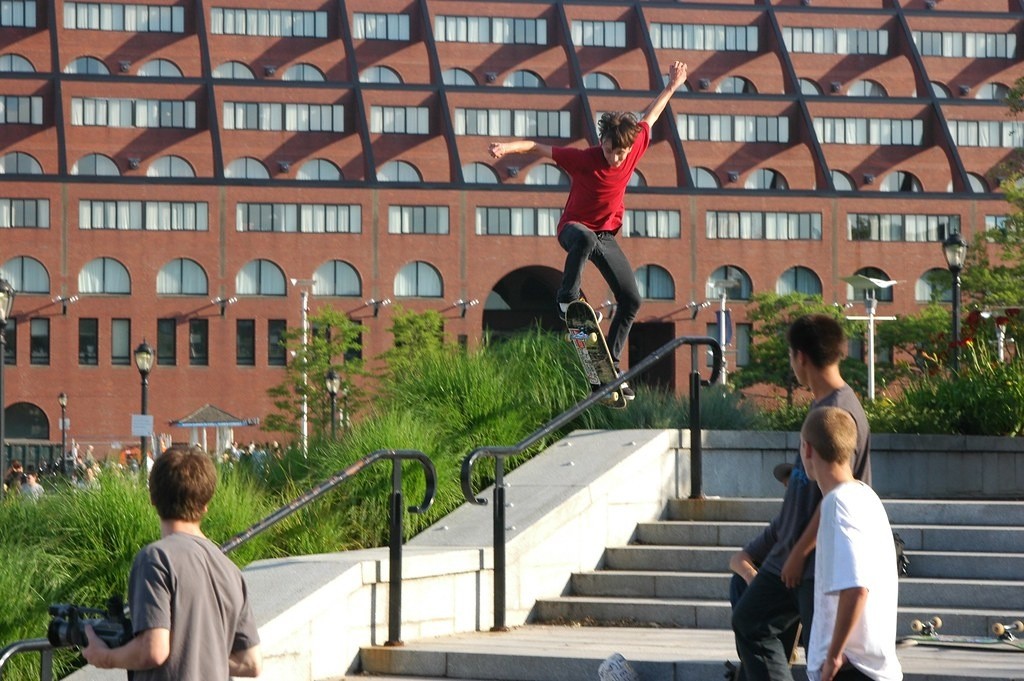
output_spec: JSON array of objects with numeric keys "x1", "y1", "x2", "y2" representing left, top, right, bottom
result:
[
  {"x1": 838, "y1": 273, "x2": 900, "y2": 401},
  {"x1": 134, "y1": 337, "x2": 155, "y2": 488},
  {"x1": 325, "y1": 370, "x2": 341, "y2": 440},
  {"x1": 942, "y1": 229, "x2": 968, "y2": 383},
  {"x1": 57, "y1": 394, "x2": 67, "y2": 479}
]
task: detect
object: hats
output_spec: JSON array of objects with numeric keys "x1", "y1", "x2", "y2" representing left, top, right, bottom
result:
[{"x1": 774, "y1": 462, "x2": 796, "y2": 484}]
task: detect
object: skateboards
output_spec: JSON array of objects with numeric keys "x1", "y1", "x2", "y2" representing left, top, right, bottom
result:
[{"x1": 562, "y1": 299, "x2": 627, "y2": 411}]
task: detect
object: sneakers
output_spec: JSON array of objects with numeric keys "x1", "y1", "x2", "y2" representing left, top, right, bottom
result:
[
  {"x1": 616, "y1": 367, "x2": 635, "y2": 401},
  {"x1": 557, "y1": 304, "x2": 603, "y2": 324}
]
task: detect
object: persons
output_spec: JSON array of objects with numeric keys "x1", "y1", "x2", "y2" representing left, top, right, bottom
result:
[
  {"x1": 0, "y1": 437, "x2": 301, "y2": 513},
  {"x1": 81, "y1": 443, "x2": 262, "y2": 681},
  {"x1": 799, "y1": 406, "x2": 904, "y2": 681},
  {"x1": 728, "y1": 313, "x2": 873, "y2": 681},
  {"x1": 488, "y1": 61, "x2": 692, "y2": 401}
]
